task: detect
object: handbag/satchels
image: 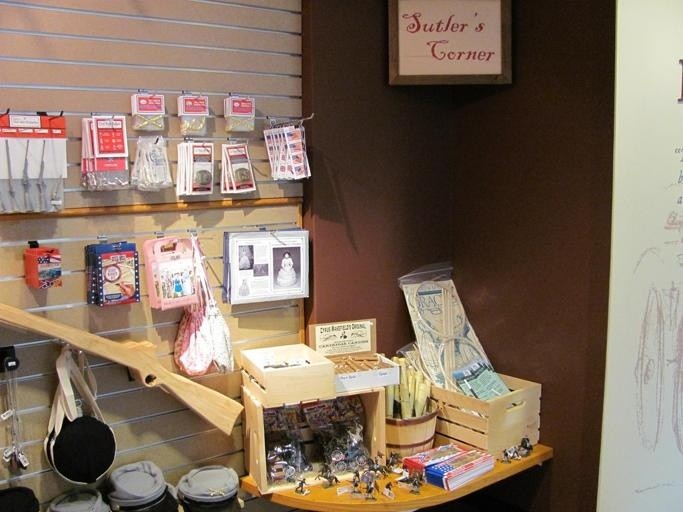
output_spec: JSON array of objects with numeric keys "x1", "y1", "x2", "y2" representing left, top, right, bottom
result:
[
  {"x1": 174, "y1": 306, "x2": 234, "y2": 377},
  {"x1": 44, "y1": 416, "x2": 117, "y2": 486}
]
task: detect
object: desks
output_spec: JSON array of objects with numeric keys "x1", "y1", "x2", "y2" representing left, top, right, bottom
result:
[{"x1": 239, "y1": 437, "x2": 553, "y2": 512}]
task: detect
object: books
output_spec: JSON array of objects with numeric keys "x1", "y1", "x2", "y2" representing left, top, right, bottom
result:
[{"x1": 401, "y1": 441, "x2": 495, "y2": 492}]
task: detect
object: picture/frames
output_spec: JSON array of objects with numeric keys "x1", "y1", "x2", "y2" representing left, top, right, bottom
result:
[{"x1": 388, "y1": 0, "x2": 512, "y2": 86}]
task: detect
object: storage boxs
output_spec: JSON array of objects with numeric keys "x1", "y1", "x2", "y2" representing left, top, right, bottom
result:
[
  {"x1": 241, "y1": 386, "x2": 386, "y2": 495},
  {"x1": 429, "y1": 374, "x2": 542, "y2": 457}
]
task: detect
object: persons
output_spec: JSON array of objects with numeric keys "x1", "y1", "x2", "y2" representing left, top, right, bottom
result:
[
  {"x1": 276, "y1": 251, "x2": 297, "y2": 287},
  {"x1": 240, "y1": 248, "x2": 251, "y2": 270}
]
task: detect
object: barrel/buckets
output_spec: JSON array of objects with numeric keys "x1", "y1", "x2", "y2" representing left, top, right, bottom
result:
[{"x1": 385, "y1": 399, "x2": 438, "y2": 462}]
